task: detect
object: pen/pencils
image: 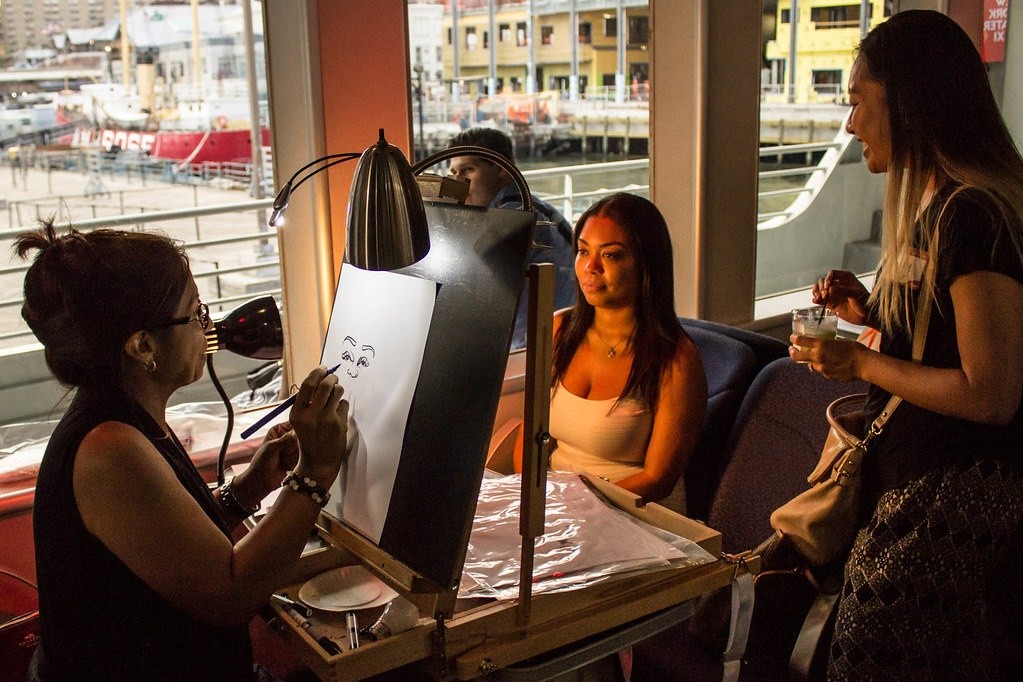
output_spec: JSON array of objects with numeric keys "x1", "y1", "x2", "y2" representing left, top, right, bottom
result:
[
  {"x1": 288, "y1": 607, "x2": 342, "y2": 656},
  {"x1": 239, "y1": 364, "x2": 342, "y2": 440},
  {"x1": 273, "y1": 595, "x2": 313, "y2": 616},
  {"x1": 345, "y1": 612, "x2": 359, "y2": 650}
]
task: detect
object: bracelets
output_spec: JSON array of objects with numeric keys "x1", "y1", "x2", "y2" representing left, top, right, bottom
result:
[
  {"x1": 220, "y1": 476, "x2": 262, "y2": 519},
  {"x1": 284, "y1": 472, "x2": 331, "y2": 508}
]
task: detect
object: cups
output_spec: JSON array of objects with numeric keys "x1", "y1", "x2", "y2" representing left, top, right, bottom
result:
[{"x1": 791, "y1": 307, "x2": 839, "y2": 364}]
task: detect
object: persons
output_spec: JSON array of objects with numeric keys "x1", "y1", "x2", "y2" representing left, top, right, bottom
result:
[
  {"x1": 12, "y1": 218, "x2": 350, "y2": 682},
  {"x1": 445, "y1": 127, "x2": 577, "y2": 352},
  {"x1": 784, "y1": 14, "x2": 1023, "y2": 682},
  {"x1": 511, "y1": 191, "x2": 707, "y2": 516}
]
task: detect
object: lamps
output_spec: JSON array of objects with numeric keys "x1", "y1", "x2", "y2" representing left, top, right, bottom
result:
[
  {"x1": 345, "y1": 127, "x2": 531, "y2": 272},
  {"x1": 204, "y1": 295, "x2": 286, "y2": 486},
  {"x1": 268, "y1": 153, "x2": 362, "y2": 229}
]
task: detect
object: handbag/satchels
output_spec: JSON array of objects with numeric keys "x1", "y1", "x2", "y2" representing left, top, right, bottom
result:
[{"x1": 770, "y1": 392, "x2": 889, "y2": 569}]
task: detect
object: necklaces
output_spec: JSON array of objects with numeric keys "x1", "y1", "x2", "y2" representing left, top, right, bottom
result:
[{"x1": 593, "y1": 326, "x2": 628, "y2": 358}]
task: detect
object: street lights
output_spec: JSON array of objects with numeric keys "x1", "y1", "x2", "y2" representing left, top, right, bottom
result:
[{"x1": 415, "y1": 60, "x2": 425, "y2": 162}]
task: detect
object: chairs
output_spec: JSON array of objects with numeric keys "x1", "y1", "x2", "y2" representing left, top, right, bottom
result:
[{"x1": 485, "y1": 305, "x2": 865, "y2": 682}]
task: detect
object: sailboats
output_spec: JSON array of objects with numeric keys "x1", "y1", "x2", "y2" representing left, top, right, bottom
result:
[{"x1": 49, "y1": 0, "x2": 273, "y2": 173}]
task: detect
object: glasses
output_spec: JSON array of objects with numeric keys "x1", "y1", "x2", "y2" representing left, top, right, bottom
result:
[{"x1": 156, "y1": 303, "x2": 210, "y2": 332}]
